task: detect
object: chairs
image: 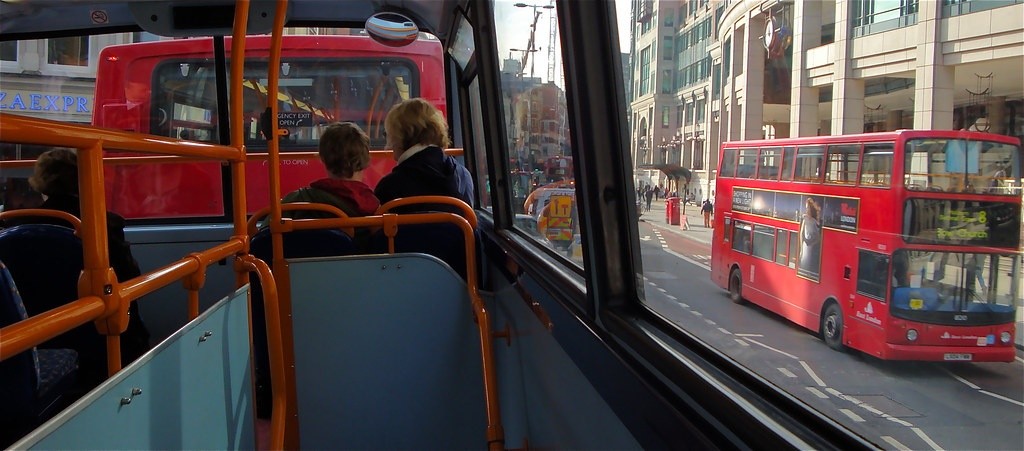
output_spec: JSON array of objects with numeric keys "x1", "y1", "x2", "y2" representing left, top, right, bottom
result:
[
  {"x1": 0, "y1": 260, "x2": 78, "y2": 403},
  {"x1": 246, "y1": 201, "x2": 355, "y2": 289},
  {"x1": 370, "y1": 196, "x2": 488, "y2": 290},
  {"x1": 0, "y1": 208, "x2": 82, "y2": 317}
]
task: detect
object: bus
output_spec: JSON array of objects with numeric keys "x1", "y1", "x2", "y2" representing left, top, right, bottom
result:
[
  {"x1": 709, "y1": 129, "x2": 1024, "y2": 363},
  {"x1": 546, "y1": 156, "x2": 573, "y2": 183},
  {"x1": 92, "y1": 34, "x2": 449, "y2": 220}
]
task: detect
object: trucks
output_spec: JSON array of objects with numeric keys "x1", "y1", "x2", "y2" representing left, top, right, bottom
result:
[{"x1": 524, "y1": 180, "x2": 576, "y2": 257}]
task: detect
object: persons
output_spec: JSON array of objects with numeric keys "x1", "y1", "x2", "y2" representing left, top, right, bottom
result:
[
  {"x1": 975, "y1": 254, "x2": 987, "y2": 294},
  {"x1": 701, "y1": 199, "x2": 713, "y2": 228},
  {"x1": 800, "y1": 197, "x2": 821, "y2": 273},
  {"x1": 308, "y1": 121, "x2": 386, "y2": 233},
  {"x1": 660, "y1": 184, "x2": 672, "y2": 199},
  {"x1": 645, "y1": 187, "x2": 653, "y2": 211},
  {"x1": 28, "y1": 148, "x2": 150, "y2": 369},
  {"x1": 645, "y1": 182, "x2": 650, "y2": 191},
  {"x1": 654, "y1": 185, "x2": 660, "y2": 201},
  {"x1": 374, "y1": 98, "x2": 475, "y2": 218},
  {"x1": 0, "y1": 176, "x2": 45, "y2": 212}
]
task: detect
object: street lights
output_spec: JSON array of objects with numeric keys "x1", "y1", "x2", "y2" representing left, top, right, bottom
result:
[
  {"x1": 510, "y1": 49, "x2": 538, "y2": 101},
  {"x1": 514, "y1": 3, "x2": 554, "y2": 173}
]
